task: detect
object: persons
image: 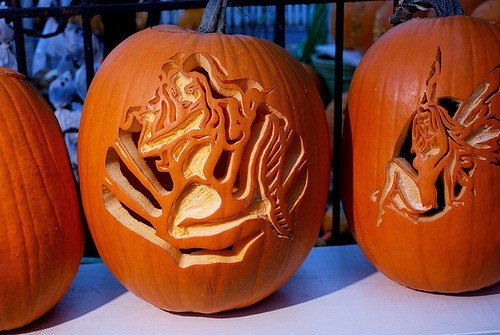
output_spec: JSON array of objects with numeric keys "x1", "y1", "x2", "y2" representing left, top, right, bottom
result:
[{"x1": 90, "y1": 0, "x2": 148, "y2": 63}]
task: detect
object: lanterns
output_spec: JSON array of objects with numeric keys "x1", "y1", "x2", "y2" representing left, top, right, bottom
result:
[
  {"x1": 336, "y1": 14, "x2": 500, "y2": 296},
  {"x1": 76, "y1": 23, "x2": 331, "y2": 315},
  {"x1": 0, "y1": 66, "x2": 85, "y2": 332}
]
